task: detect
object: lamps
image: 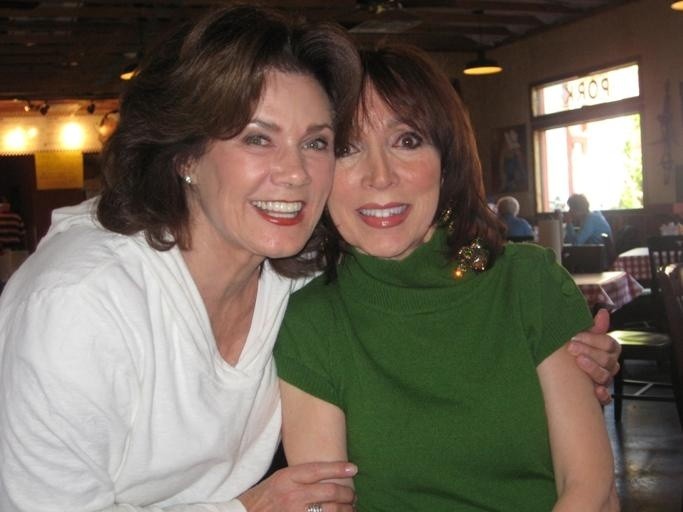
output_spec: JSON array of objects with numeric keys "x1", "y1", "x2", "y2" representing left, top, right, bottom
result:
[
  {"x1": 118, "y1": 18, "x2": 145, "y2": 81},
  {"x1": 460, "y1": 8, "x2": 503, "y2": 77}
]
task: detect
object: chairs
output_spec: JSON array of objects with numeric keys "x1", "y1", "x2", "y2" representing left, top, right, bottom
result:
[{"x1": 501, "y1": 224, "x2": 682, "y2": 422}]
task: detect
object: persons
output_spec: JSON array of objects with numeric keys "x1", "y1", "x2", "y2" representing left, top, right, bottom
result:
[
  {"x1": 494, "y1": 193, "x2": 534, "y2": 241},
  {"x1": 0, "y1": 1, "x2": 620, "y2": 511},
  {"x1": 269, "y1": 37, "x2": 628, "y2": 510},
  {"x1": 0, "y1": 199, "x2": 27, "y2": 277},
  {"x1": 559, "y1": 194, "x2": 616, "y2": 272},
  {"x1": 82, "y1": 172, "x2": 107, "y2": 198}
]
